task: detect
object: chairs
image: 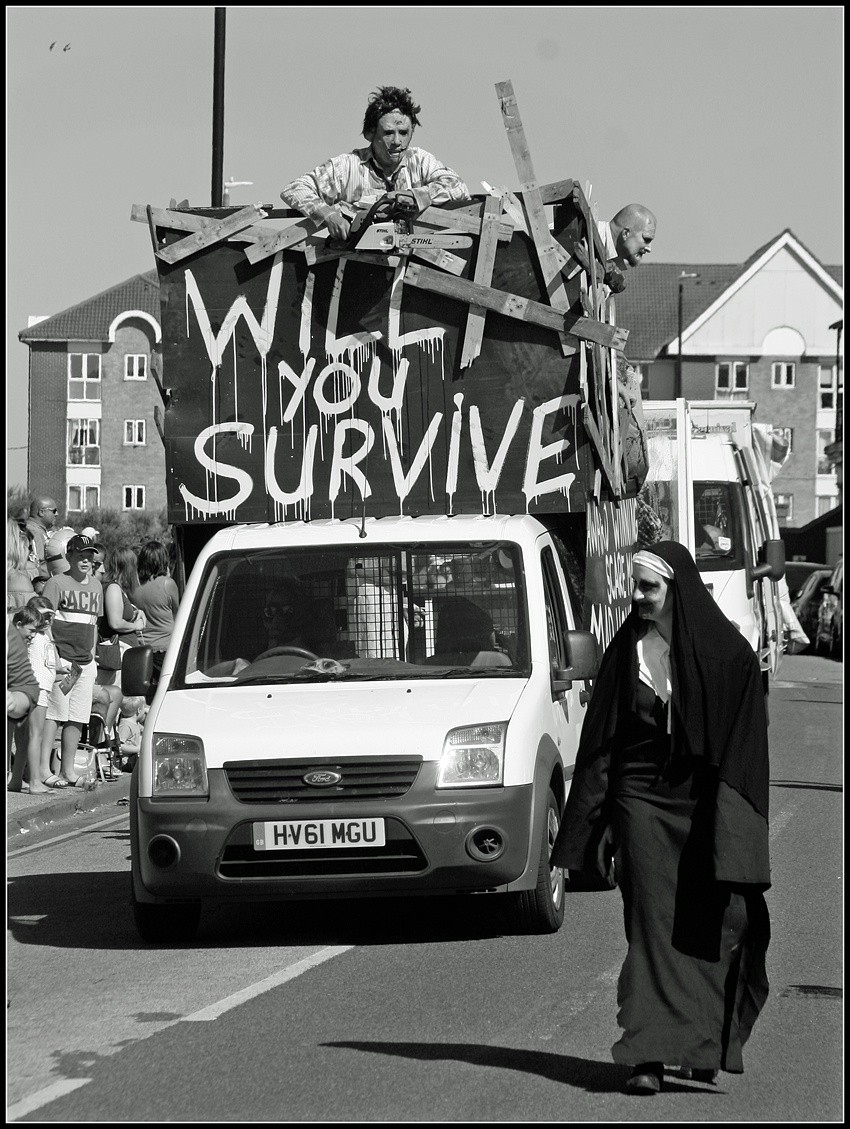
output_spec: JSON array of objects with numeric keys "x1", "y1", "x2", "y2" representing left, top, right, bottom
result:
[{"x1": 424, "y1": 611, "x2": 498, "y2": 667}]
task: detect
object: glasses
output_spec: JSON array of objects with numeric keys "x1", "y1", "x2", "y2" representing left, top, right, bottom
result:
[{"x1": 43, "y1": 508, "x2": 57, "y2": 514}]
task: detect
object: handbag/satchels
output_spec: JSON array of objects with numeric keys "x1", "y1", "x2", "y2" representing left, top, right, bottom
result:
[{"x1": 98, "y1": 644, "x2": 122, "y2": 670}]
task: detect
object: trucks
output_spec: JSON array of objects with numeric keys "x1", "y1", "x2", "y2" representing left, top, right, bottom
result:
[
  {"x1": 631, "y1": 398, "x2": 785, "y2": 692},
  {"x1": 106, "y1": 491, "x2": 640, "y2": 933}
]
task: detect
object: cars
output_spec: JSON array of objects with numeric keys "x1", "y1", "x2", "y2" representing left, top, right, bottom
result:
[
  {"x1": 784, "y1": 562, "x2": 830, "y2": 600},
  {"x1": 815, "y1": 558, "x2": 843, "y2": 656},
  {"x1": 790, "y1": 570, "x2": 834, "y2": 634}
]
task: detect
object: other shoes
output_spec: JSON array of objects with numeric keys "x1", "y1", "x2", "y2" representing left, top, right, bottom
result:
[{"x1": 626, "y1": 1062, "x2": 664, "y2": 1091}]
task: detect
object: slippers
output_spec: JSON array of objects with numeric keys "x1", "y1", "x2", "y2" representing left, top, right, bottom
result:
[
  {"x1": 43, "y1": 775, "x2": 69, "y2": 788},
  {"x1": 68, "y1": 776, "x2": 85, "y2": 787}
]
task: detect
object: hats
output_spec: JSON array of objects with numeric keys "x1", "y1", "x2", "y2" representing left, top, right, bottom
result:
[{"x1": 67, "y1": 534, "x2": 99, "y2": 554}]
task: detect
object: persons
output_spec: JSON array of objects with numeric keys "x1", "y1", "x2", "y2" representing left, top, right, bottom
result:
[
  {"x1": 96, "y1": 547, "x2": 147, "y2": 744},
  {"x1": 583, "y1": 204, "x2": 657, "y2": 262},
  {"x1": 280, "y1": 85, "x2": 472, "y2": 240},
  {"x1": 39, "y1": 535, "x2": 104, "y2": 788},
  {"x1": 423, "y1": 595, "x2": 496, "y2": 665},
  {"x1": 252, "y1": 584, "x2": 339, "y2": 662},
  {"x1": 27, "y1": 597, "x2": 56, "y2": 794},
  {"x1": 6, "y1": 496, "x2": 123, "y2": 793},
  {"x1": 550, "y1": 540, "x2": 772, "y2": 1091},
  {"x1": 118, "y1": 696, "x2": 145, "y2": 773},
  {"x1": 128, "y1": 541, "x2": 179, "y2": 682}
]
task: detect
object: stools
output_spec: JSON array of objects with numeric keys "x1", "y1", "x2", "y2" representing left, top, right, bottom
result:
[{"x1": 85, "y1": 712, "x2": 124, "y2": 781}]
